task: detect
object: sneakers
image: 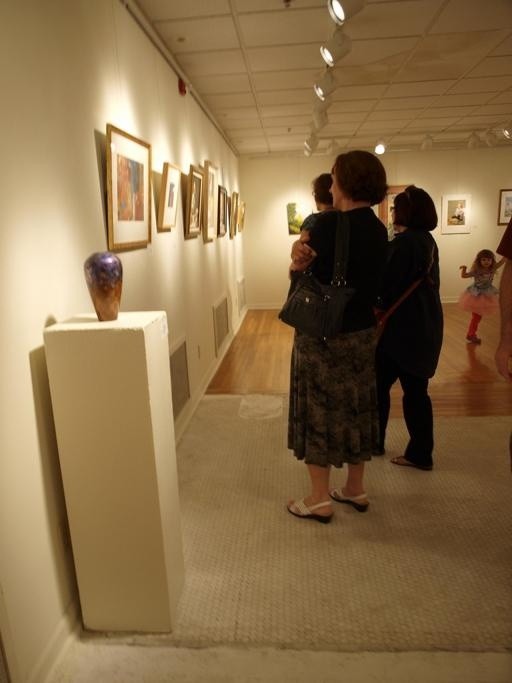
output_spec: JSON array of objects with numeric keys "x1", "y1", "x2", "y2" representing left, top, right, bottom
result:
[{"x1": 466, "y1": 334, "x2": 480, "y2": 342}]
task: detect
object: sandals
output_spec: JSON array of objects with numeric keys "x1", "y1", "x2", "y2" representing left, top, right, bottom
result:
[
  {"x1": 287, "y1": 498, "x2": 336, "y2": 523},
  {"x1": 392, "y1": 456, "x2": 415, "y2": 466},
  {"x1": 330, "y1": 486, "x2": 369, "y2": 511}
]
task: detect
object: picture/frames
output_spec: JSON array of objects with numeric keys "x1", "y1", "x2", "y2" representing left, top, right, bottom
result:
[
  {"x1": 107, "y1": 121, "x2": 246, "y2": 249},
  {"x1": 378, "y1": 185, "x2": 512, "y2": 241}
]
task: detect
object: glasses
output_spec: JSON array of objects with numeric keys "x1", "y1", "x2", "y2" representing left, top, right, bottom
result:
[{"x1": 390, "y1": 206, "x2": 396, "y2": 212}]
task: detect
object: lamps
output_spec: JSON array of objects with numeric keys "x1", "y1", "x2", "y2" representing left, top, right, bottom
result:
[{"x1": 312, "y1": 0, "x2": 365, "y2": 102}]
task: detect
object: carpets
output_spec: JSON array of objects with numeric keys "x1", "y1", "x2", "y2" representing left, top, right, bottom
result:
[{"x1": 77, "y1": 393, "x2": 510, "y2": 650}]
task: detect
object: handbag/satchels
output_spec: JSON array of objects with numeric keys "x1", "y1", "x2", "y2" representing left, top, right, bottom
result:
[{"x1": 277, "y1": 273, "x2": 354, "y2": 342}]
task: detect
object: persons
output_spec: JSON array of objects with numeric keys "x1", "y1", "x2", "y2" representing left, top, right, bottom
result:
[
  {"x1": 457, "y1": 246, "x2": 506, "y2": 345},
  {"x1": 493, "y1": 216, "x2": 512, "y2": 383},
  {"x1": 371, "y1": 185, "x2": 445, "y2": 472},
  {"x1": 453, "y1": 203, "x2": 465, "y2": 221},
  {"x1": 278, "y1": 150, "x2": 390, "y2": 526},
  {"x1": 287, "y1": 172, "x2": 338, "y2": 303}
]
